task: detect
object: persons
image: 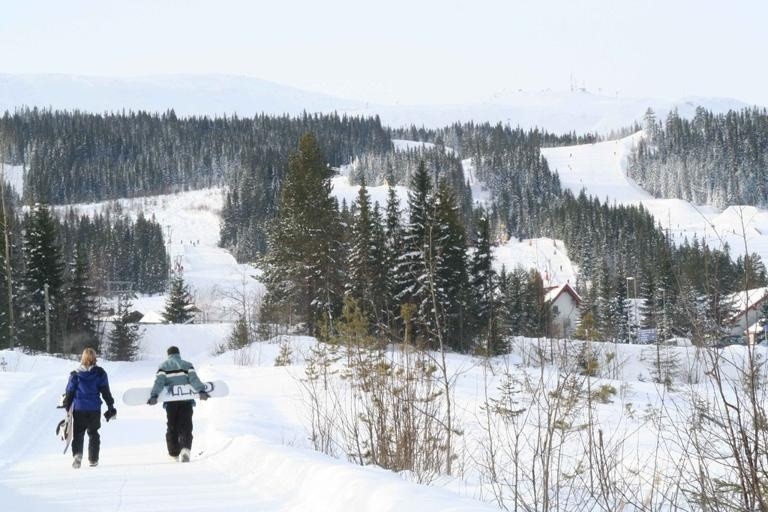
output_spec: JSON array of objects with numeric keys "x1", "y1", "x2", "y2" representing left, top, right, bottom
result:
[
  {"x1": 61, "y1": 346, "x2": 117, "y2": 470},
  {"x1": 146, "y1": 345, "x2": 213, "y2": 465}
]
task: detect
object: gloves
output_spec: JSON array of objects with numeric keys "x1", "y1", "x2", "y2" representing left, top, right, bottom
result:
[
  {"x1": 197, "y1": 388, "x2": 211, "y2": 400},
  {"x1": 147, "y1": 393, "x2": 158, "y2": 405}
]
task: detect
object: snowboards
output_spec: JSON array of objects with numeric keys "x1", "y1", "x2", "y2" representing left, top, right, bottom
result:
[
  {"x1": 123, "y1": 380, "x2": 229, "y2": 406},
  {"x1": 57, "y1": 393, "x2": 74, "y2": 455}
]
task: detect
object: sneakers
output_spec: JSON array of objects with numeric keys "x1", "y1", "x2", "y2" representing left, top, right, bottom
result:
[
  {"x1": 181, "y1": 448, "x2": 190, "y2": 462},
  {"x1": 170, "y1": 455, "x2": 179, "y2": 463},
  {"x1": 72, "y1": 452, "x2": 82, "y2": 468},
  {"x1": 89, "y1": 460, "x2": 98, "y2": 466}
]
